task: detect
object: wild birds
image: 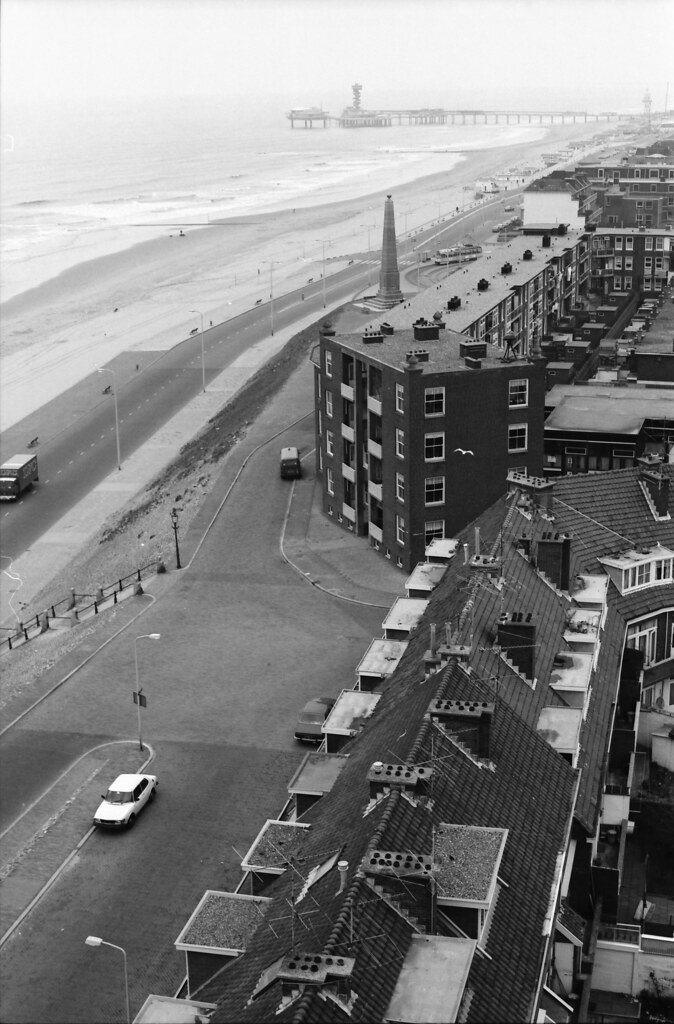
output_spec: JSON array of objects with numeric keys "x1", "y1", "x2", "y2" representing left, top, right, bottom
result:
[{"x1": 455, "y1": 449, "x2": 474, "y2": 456}]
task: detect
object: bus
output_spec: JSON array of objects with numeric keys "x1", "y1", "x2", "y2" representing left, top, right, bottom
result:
[
  {"x1": 280, "y1": 447, "x2": 301, "y2": 481},
  {"x1": 434, "y1": 245, "x2": 482, "y2": 266}
]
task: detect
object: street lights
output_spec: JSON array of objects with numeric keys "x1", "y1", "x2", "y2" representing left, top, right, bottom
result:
[
  {"x1": 261, "y1": 259, "x2": 274, "y2": 336},
  {"x1": 134, "y1": 633, "x2": 161, "y2": 751},
  {"x1": 169, "y1": 508, "x2": 182, "y2": 570},
  {"x1": 189, "y1": 309, "x2": 206, "y2": 392},
  {"x1": 96, "y1": 368, "x2": 121, "y2": 471},
  {"x1": 316, "y1": 238, "x2": 332, "y2": 310}
]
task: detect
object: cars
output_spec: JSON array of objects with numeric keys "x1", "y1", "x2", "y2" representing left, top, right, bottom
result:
[
  {"x1": 294, "y1": 696, "x2": 337, "y2": 745},
  {"x1": 92, "y1": 773, "x2": 159, "y2": 833},
  {"x1": 492, "y1": 215, "x2": 520, "y2": 234},
  {"x1": 504, "y1": 206, "x2": 514, "y2": 212}
]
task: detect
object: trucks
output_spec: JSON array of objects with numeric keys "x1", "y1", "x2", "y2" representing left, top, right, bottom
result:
[{"x1": 0, "y1": 452, "x2": 40, "y2": 503}]
